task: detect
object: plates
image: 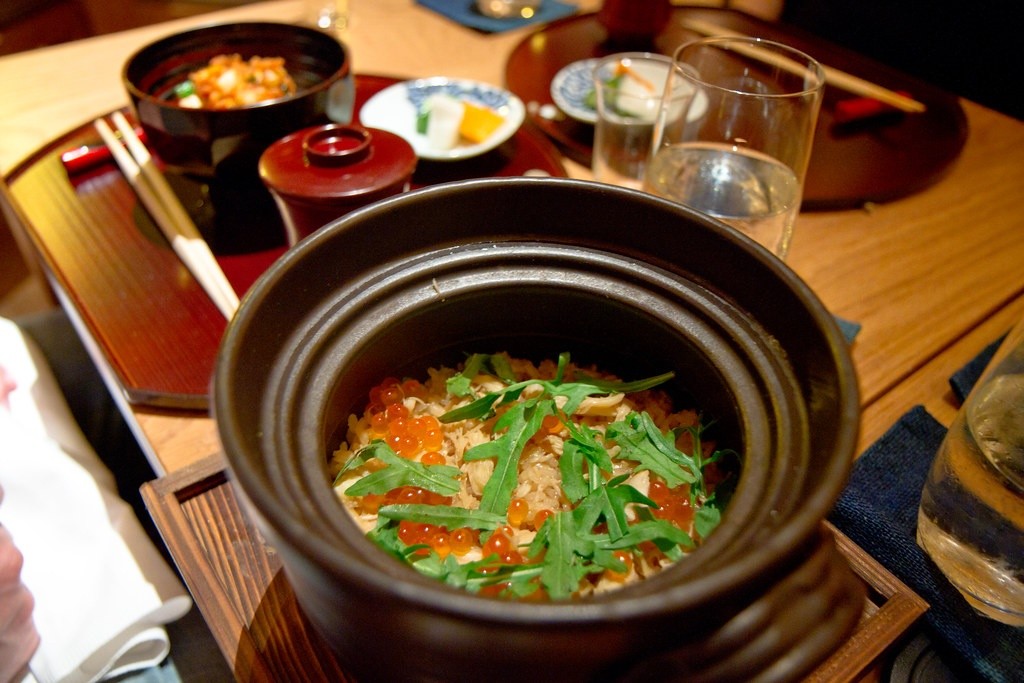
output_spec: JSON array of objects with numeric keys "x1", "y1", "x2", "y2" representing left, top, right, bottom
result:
[
  {"x1": 357, "y1": 76, "x2": 527, "y2": 163},
  {"x1": 551, "y1": 57, "x2": 709, "y2": 132}
]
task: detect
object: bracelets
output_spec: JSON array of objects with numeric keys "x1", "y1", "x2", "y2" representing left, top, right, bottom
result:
[{"x1": 722, "y1": 0, "x2": 728, "y2": 10}]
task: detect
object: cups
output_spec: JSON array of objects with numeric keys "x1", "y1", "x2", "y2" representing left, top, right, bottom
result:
[
  {"x1": 593, "y1": 52, "x2": 702, "y2": 184},
  {"x1": 914, "y1": 322, "x2": 1023, "y2": 633},
  {"x1": 299, "y1": 0, "x2": 348, "y2": 37},
  {"x1": 642, "y1": 34, "x2": 824, "y2": 265},
  {"x1": 476, "y1": 0, "x2": 539, "y2": 19}
]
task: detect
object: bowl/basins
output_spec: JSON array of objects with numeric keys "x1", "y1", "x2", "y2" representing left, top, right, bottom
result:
[
  {"x1": 121, "y1": 20, "x2": 351, "y2": 179},
  {"x1": 210, "y1": 170, "x2": 865, "y2": 681}
]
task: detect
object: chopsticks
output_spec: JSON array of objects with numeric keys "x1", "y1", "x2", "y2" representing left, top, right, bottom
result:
[
  {"x1": 96, "y1": 111, "x2": 242, "y2": 324},
  {"x1": 683, "y1": 18, "x2": 925, "y2": 113}
]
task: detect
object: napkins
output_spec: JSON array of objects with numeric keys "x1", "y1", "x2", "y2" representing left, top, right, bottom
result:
[{"x1": 0, "y1": 316, "x2": 193, "y2": 683}]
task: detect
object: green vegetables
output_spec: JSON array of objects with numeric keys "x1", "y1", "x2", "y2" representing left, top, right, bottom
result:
[{"x1": 332, "y1": 354, "x2": 734, "y2": 598}]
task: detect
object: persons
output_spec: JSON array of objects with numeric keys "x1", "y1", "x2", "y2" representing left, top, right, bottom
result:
[
  {"x1": 671, "y1": 0, "x2": 782, "y2": 24},
  {"x1": 0, "y1": 362, "x2": 43, "y2": 683}
]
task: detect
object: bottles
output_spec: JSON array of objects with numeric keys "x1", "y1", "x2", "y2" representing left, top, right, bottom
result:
[{"x1": 258, "y1": 123, "x2": 417, "y2": 254}]
